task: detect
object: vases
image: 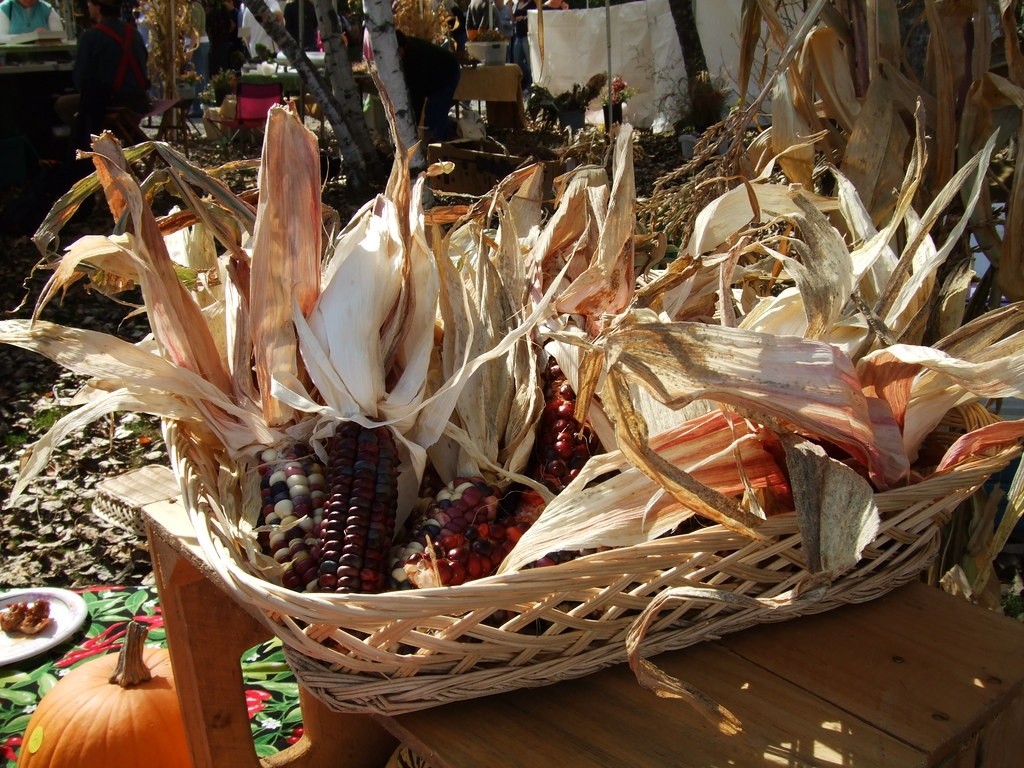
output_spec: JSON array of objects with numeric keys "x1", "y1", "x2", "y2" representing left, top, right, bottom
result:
[{"x1": 604, "y1": 105, "x2": 622, "y2": 133}]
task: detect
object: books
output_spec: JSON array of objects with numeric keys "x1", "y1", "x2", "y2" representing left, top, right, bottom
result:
[{"x1": 11, "y1": 31, "x2": 64, "y2": 44}]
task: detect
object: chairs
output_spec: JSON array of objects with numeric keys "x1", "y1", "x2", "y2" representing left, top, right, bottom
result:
[{"x1": 207, "y1": 83, "x2": 282, "y2": 159}]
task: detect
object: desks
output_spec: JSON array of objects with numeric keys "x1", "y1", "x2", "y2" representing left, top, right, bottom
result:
[
  {"x1": 452, "y1": 64, "x2": 527, "y2": 130},
  {"x1": 144, "y1": 520, "x2": 1023, "y2": 768},
  {"x1": 243, "y1": 73, "x2": 378, "y2": 113}
]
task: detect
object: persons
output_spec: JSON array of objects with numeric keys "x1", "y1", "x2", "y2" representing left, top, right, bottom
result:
[
  {"x1": 440, "y1": 0, "x2": 569, "y2": 94},
  {"x1": 395, "y1": 30, "x2": 461, "y2": 134},
  {"x1": 184, "y1": 0, "x2": 349, "y2": 118},
  {"x1": 0, "y1": 0, "x2": 66, "y2": 46},
  {"x1": 54, "y1": 0, "x2": 164, "y2": 137}
]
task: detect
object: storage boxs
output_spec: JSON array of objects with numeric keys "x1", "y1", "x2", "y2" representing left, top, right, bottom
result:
[{"x1": 465, "y1": 41, "x2": 509, "y2": 64}]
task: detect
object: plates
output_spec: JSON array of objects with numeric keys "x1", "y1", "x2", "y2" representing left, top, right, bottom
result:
[{"x1": 0, "y1": 587, "x2": 88, "y2": 667}]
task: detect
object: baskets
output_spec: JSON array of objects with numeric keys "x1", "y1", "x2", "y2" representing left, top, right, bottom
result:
[{"x1": 161, "y1": 415, "x2": 1024, "y2": 717}]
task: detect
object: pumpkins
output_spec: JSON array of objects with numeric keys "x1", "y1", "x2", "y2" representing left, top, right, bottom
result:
[{"x1": 14, "y1": 620, "x2": 197, "y2": 768}]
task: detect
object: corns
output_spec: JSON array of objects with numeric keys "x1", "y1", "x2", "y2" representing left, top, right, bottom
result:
[{"x1": 250, "y1": 362, "x2": 608, "y2": 665}]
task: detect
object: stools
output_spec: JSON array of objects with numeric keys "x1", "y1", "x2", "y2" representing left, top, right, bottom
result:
[{"x1": 106, "y1": 99, "x2": 193, "y2": 180}]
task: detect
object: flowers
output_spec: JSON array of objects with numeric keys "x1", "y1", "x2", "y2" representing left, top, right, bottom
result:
[
  {"x1": 601, "y1": 73, "x2": 637, "y2": 106},
  {"x1": 468, "y1": 28, "x2": 509, "y2": 42}
]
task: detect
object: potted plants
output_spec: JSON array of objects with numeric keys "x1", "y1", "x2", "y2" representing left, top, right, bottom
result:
[
  {"x1": 526, "y1": 71, "x2": 608, "y2": 134},
  {"x1": 660, "y1": 69, "x2": 734, "y2": 158},
  {"x1": 198, "y1": 66, "x2": 235, "y2": 137}
]
